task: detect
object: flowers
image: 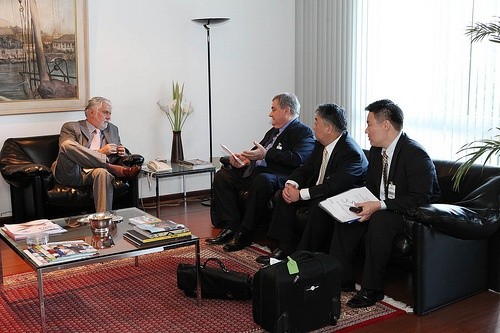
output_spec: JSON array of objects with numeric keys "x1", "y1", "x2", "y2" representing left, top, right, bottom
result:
[{"x1": 155, "y1": 78, "x2": 195, "y2": 130}]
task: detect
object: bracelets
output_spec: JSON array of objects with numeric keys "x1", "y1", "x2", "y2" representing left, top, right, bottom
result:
[{"x1": 299, "y1": 193, "x2": 303, "y2": 200}]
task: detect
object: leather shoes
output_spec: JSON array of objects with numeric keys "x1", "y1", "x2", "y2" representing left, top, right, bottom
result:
[
  {"x1": 256, "y1": 248, "x2": 287, "y2": 264},
  {"x1": 346, "y1": 289, "x2": 384, "y2": 308},
  {"x1": 223, "y1": 233, "x2": 251, "y2": 251},
  {"x1": 205, "y1": 227, "x2": 235, "y2": 243}
]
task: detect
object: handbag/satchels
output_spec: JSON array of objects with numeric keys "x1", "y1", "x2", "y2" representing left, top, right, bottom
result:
[
  {"x1": 252, "y1": 250, "x2": 340, "y2": 333},
  {"x1": 176, "y1": 258, "x2": 251, "y2": 301}
]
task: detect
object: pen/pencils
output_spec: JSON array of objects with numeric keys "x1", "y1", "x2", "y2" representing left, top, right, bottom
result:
[{"x1": 344, "y1": 202, "x2": 356, "y2": 207}]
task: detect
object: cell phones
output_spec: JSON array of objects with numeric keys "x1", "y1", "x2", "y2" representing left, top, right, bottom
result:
[{"x1": 349, "y1": 206, "x2": 363, "y2": 213}]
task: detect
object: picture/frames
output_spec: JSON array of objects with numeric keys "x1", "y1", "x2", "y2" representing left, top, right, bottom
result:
[{"x1": 0, "y1": 0, "x2": 92, "y2": 116}]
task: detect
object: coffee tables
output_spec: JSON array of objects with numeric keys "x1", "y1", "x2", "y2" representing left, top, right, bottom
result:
[{"x1": 0, "y1": 206, "x2": 203, "y2": 333}]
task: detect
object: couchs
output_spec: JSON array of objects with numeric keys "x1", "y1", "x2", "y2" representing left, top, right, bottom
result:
[
  {"x1": 208, "y1": 150, "x2": 500, "y2": 316},
  {"x1": 0, "y1": 134, "x2": 145, "y2": 224}
]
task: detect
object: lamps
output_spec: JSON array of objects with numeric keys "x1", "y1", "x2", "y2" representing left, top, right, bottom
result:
[{"x1": 191, "y1": 17, "x2": 230, "y2": 162}]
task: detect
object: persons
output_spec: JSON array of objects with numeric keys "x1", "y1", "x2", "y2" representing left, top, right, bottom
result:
[
  {"x1": 204, "y1": 94, "x2": 315, "y2": 251},
  {"x1": 329, "y1": 99, "x2": 442, "y2": 308},
  {"x1": 255, "y1": 103, "x2": 370, "y2": 265},
  {"x1": 53, "y1": 96, "x2": 140, "y2": 212}
]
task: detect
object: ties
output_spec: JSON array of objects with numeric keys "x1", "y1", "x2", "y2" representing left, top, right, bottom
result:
[
  {"x1": 242, "y1": 130, "x2": 278, "y2": 178},
  {"x1": 382, "y1": 151, "x2": 388, "y2": 200},
  {"x1": 83, "y1": 129, "x2": 100, "y2": 173},
  {"x1": 316, "y1": 150, "x2": 328, "y2": 186}
]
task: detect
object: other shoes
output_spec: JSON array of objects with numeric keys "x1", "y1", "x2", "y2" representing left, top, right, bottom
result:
[{"x1": 123, "y1": 166, "x2": 141, "y2": 176}]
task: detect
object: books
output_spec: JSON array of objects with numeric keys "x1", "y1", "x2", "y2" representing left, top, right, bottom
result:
[
  {"x1": 2, "y1": 216, "x2": 192, "y2": 267},
  {"x1": 179, "y1": 158, "x2": 213, "y2": 170}
]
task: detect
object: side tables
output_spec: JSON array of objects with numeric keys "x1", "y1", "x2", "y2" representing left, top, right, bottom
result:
[{"x1": 141, "y1": 160, "x2": 217, "y2": 218}]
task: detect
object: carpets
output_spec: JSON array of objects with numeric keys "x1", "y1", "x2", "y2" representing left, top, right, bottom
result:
[{"x1": 0, "y1": 230, "x2": 414, "y2": 333}]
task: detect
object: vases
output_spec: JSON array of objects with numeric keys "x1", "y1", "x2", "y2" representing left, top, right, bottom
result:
[{"x1": 171, "y1": 130, "x2": 184, "y2": 162}]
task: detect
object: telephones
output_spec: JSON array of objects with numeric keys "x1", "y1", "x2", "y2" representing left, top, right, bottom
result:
[{"x1": 146, "y1": 160, "x2": 173, "y2": 172}]
